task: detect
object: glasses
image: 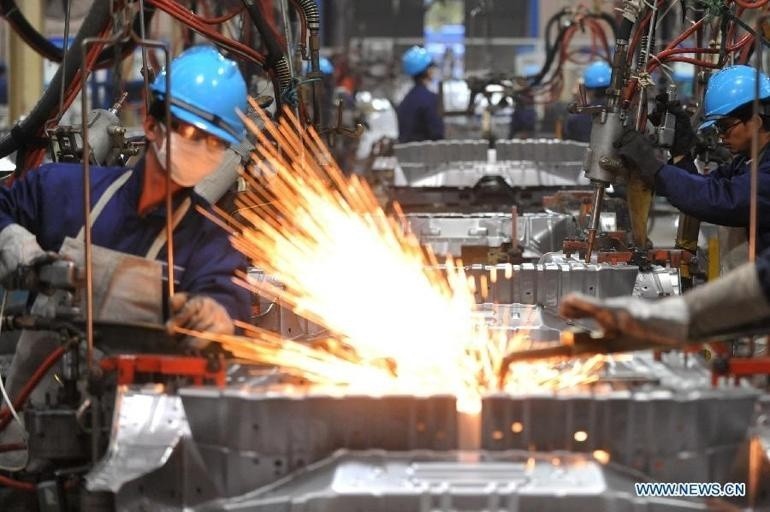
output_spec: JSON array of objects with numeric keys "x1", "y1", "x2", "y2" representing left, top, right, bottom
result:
[
  {"x1": 165, "y1": 117, "x2": 230, "y2": 150},
  {"x1": 712, "y1": 121, "x2": 744, "y2": 134}
]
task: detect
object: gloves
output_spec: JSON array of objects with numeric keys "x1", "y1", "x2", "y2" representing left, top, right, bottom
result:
[
  {"x1": 648, "y1": 95, "x2": 699, "y2": 156},
  {"x1": 559, "y1": 264, "x2": 769, "y2": 350},
  {"x1": 167, "y1": 292, "x2": 234, "y2": 349},
  {"x1": 0, "y1": 224, "x2": 43, "y2": 278},
  {"x1": 619, "y1": 137, "x2": 665, "y2": 185}
]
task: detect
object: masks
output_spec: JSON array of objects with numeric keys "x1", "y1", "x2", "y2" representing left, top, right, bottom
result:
[{"x1": 151, "y1": 120, "x2": 224, "y2": 188}]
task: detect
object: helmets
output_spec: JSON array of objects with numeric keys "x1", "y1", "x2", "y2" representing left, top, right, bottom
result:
[
  {"x1": 402, "y1": 46, "x2": 432, "y2": 77},
  {"x1": 699, "y1": 65, "x2": 770, "y2": 131},
  {"x1": 584, "y1": 61, "x2": 613, "y2": 88},
  {"x1": 149, "y1": 45, "x2": 248, "y2": 144}
]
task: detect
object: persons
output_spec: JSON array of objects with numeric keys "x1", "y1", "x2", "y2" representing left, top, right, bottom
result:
[
  {"x1": 508, "y1": 89, "x2": 568, "y2": 139},
  {"x1": 558, "y1": 246, "x2": 770, "y2": 348},
  {"x1": 398, "y1": 47, "x2": 444, "y2": 143},
  {"x1": 0, "y1": 46, "x2": 251, "y2": 512},
  {"x1": 565, "y1": 61, "x2": 612, "y2": 142},
  {"x1": 614, "y1": 65, "x2": 770, "y2": 257},
  {"x1": 301, "y1": 57, "x2": 354, "y2": 109}
]
